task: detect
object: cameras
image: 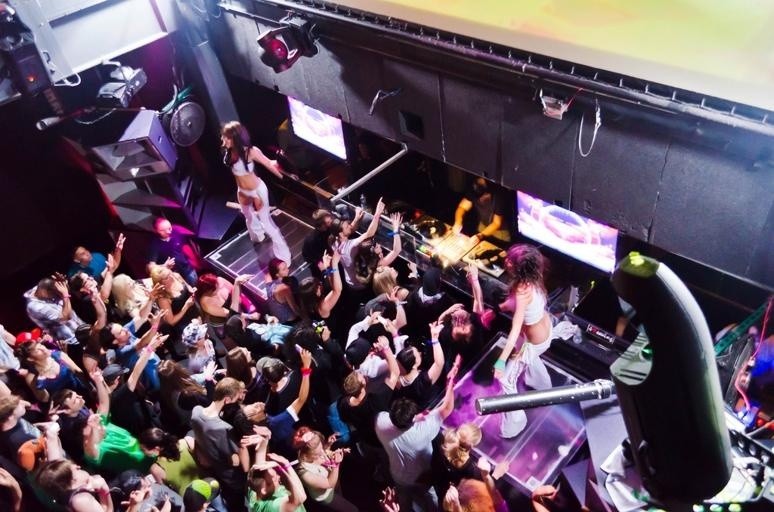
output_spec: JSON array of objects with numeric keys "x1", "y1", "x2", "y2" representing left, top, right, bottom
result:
[
  {"x1": 312, "y1": 319, "x2": 325, "y2": 330},
  {"x1": 295, "y1": 343, "x2": 304, "y2": 353}
]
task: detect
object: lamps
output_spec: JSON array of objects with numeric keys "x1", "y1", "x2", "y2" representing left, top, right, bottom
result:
[{"x1": 257, "y1": 14, "x2": 319, "y2": 74}]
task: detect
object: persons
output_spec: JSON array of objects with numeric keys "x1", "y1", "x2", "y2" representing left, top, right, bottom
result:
[
  {"x1": 531, "y1": 480, "x2": 581, "y2": 512},
  {"x1": 220, "y1": 122, "x2": 292, "y2": 263},
  {"x1": 481, "y1": 244, "x2": 553, "y2": 439},
  {"x1": 1, "y1": 197, "x2": 508, "y2": 512},
  {"x1": 451, "y1": 176, "x2": 510, "y2": 243}
]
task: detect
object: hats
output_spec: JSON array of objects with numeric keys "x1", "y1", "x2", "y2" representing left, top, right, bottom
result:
[
  {"x1": 346, "y1": 337, "x2": 370, "y2": 368},
  {"x1": 102, "y1": 364, "x2": 130, "y2": 378},
  {"x1": 183, "y1": 476, "x2": 221, "y2": 510},
  {"x1": 12, "y1": 327, "x2": 41, "y2": 347},
  {"x1": 255, "y1": 356, "x2": 287, "y2": 383}
]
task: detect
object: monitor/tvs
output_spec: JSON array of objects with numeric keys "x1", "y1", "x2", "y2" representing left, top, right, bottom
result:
[
  {"x1": 513, "y1": 188, "x2": 620, "y2": 277},
  {"x1": 379, "y1": 214, "x2": 422, "y2": 247},
  {"x1": 287, "y1": 98, "x2": 351, "y2": 162}
]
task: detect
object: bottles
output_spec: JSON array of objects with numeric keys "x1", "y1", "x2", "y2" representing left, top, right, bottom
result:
[
  {"x1": 573, "y1": 327, "x2": 583, "y2": 344},
  {"x1": 360, "y1": 194, "x2": 372, "y2": 216},
  {"x1": 568, "y1": 282, "x2": 579, "y2": 312}
]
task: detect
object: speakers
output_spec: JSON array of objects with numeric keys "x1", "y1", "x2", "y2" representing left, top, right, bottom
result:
[
  {"x1": 6, "y1": 41, "x2": 54, "y2": 98},
  {"x1": 93, "y1": 160, "x2": 210, "y2": 238},
  {"x1": 91, "y1": 110, "x2": 178, "y2": 181}
]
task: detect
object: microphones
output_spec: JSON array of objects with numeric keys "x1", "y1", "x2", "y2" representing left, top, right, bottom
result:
[{"x1": 368, "y1": 94, "x2": 380, "y2": 116}]
